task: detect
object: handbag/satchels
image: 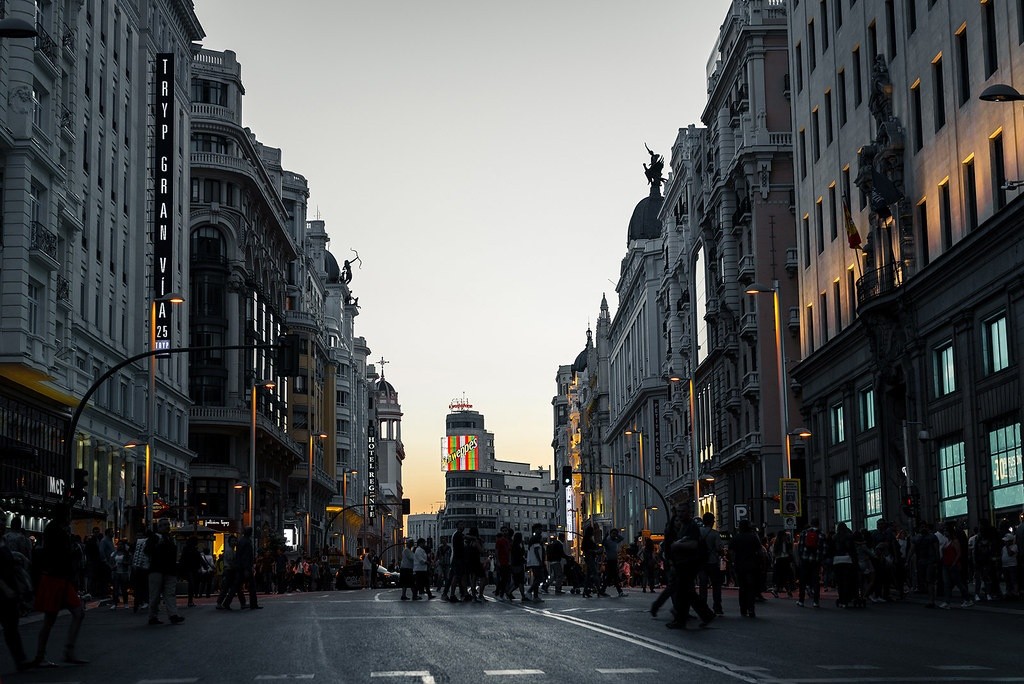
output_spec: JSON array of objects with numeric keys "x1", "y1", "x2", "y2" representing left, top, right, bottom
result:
[{"x1": 133, "y1": 539, "x2": 151, "y2": 569}]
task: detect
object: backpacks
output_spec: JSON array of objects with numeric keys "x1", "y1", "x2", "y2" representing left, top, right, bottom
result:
[
  {"x1": 700, "y1": 529, "x2": 716, "y2": 561},
  {"x1": 805, "y1": 529, "x2": 818, "y2": 548}
]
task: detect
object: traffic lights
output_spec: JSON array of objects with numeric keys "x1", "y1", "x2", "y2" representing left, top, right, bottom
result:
[
  {"x1": 74, "y1": 469, "x2": 89, "y2": 499},
  {"x1": 532, "y1": 526, "x2": 542, "y2": 538},
  {"x1": 562, "y1": 466, "x2": 572, "y2": 485},
  {"x1": 902, "y1": 484, "x2": 921, "y2": 518}
]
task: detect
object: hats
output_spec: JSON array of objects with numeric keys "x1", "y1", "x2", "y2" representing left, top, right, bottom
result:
[{"x1": 1002, "y1": 533, "x2": 1014, "y2": 541}]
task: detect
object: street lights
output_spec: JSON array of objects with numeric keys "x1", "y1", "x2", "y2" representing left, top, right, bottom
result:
[
  {"x1": 394, "y1": 527, "x2": 403, "y2": 568},
  {"x1": 296, "y1": 431, "x2": 328, "y2": 556},
  {"x1": 233, "y1": 379, "x2": 275, "y2": 554},
  {"x1": 670, "y1": 373, "x2": 715, "y2": 516},
  {"x1": 123, "y1": 291, "x2": 185, "y2": 529},
  {"x1": 381, "y1": 513, "x2": 392, "y2": 558},
  {"x1": 598, "y1": 465, "x2": 624, "y2": 535},
  {"x1": 557, "y1": 524, "x2": 566, "y2": 540},
  {"x1": 333, "y1": 469, "x2": 357, "y2": 555},
  {"x1": 747, "y1": 281, "x2": 811, "y2": 539},
  {"x1": 624, "y1": 427, "x2": 658, "y2": 529}
]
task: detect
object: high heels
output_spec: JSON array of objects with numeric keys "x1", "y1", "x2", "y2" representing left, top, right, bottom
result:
[{"x1": 583, "y1": 592, "x2": 592, "y2": 598}]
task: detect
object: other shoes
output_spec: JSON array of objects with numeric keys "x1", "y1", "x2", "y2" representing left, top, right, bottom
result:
[
  {"x1": 250, "y1": 604, "x2": 264, "y2": 609},
  {"x1": 149, "y1": 614, "x2": 185, "y2": 624},
  {"x1": 652, "y1": 601, "x2": 662, "y2": 617},
  {"x1": 665, "y1": 612, "x2": 717, "y2": 629},
  {"x1": 17, "y1": 659, "x2": 37, "y2": 672},
  {"x1": 108, "y1": 604, "x2": 129, "y2": 610},
  {"x1": 541, "y1": 585, "x2": 549, "y2": 593},
  {"x1": 555, "y1": 590, "x2": 566, "y2": 596},
  {"x1": 618, "y1": 593, "x2": 628, "y2": 597},
  {"x1": 401, "y1": 595, "x2": 410, "y2": 600},
  {"x1": 533, "y1": 596, "x2": 545, "y2": 602},
  {"x1": 187, "y1": 603, "x2": 197, "y2": 608},
  {"x1": 419, "y1": 591, "x2": 427, "y2": 594},
  {"x1": 570, "y1": 589, "x2": 583, "y2": 597},
  {"x1": 796, "y1": 601, "x2": 819, "y2": 608},
  {"x1": 141, "y1": 603, "x2": 149, "y2": 608},
  {"x1": 31, "y1": 655, "x2": 60, "y2": 667},
  {"x1": 428, "y1": 594, "x2": 436, "y2": 600},
  {"x1": 597, "y1": 591, "x2": 610, "y2": 598},
  {"x1": 755, "y1": 594, "x2": 768, "y2": 602},
  {"x1": 642, "y1": 588, "x2": 655, "y2": 593},
  {"x1": 771, "y1": 589, "x2": 793, "y2": 598},
  {"x1": 65, "y1": 656, "x2": 91, "y2": 664},
  {"x1": 241, "y1": 603, "x2": 250, "y2": 609},
  {"x1": 222, "y1": 605, "x2": 232, "y2": 610},
  {"x1": 216, "y1": 603, "x2": 223, "y2": 610},
  {"x1": 939, "y1": 600, "x2": 974, "y2": 610},
  {"x1": 442, "y1": 594, "x2": 488, "y2": 603},
  {"x1": 975, "y1": 593, "x2": 993, "y2": 601},
  {"x1": 412, "y1": 595, "x2": 423, "y2": 600},
  {"x1": 525, "y1": 591, "x2": 534, "y2": 601}
]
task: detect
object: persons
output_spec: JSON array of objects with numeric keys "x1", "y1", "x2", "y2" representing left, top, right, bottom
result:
[
  {"x1": 650, "y1": 512, "x2": 1024, "y2": 630},
  {"x1": 276, "y1": 548, "x2": 401, "y2": 590},
  {"x1": 0, "y1": 502, "x2": 89, "y2": 671},
  {"x1": 87, "y1": 518, "x2": 263, "y2": 626},
  {"x1": 401, "y1": 519, "x2": 655, "y2": 603}
]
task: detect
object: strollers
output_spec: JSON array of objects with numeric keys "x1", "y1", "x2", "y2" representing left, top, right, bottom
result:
[
  {"x1": 833, "y1": 553, "x2": 868, "y2": 608},
  {"x1": 565, "y1": 556, "x2": 597, "y2": 594}
]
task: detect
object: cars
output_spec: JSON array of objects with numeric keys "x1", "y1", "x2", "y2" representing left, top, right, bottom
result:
[{"x1": 344, "y1": 563, "x2": 400, "y2": 587}]
